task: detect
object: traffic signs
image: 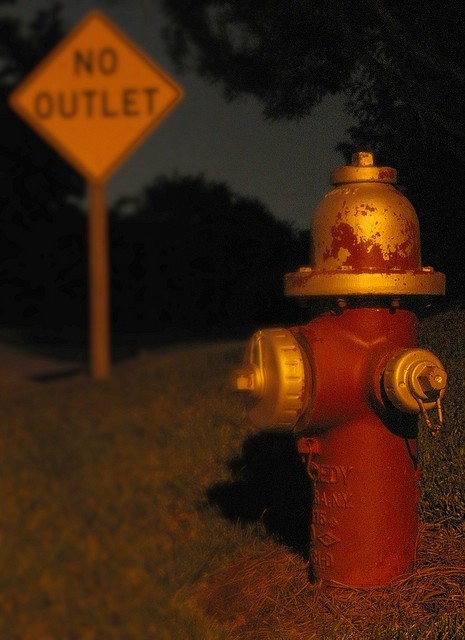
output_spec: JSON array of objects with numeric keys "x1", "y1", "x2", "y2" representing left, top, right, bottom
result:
[{"x1": 11, "y1": 11, "x2": 184, "y2": 383}]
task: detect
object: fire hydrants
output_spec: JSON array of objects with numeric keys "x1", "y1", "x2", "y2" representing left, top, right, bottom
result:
[{"x1": 234, "y1": 147, "x2": 449, "y2": 592}]
